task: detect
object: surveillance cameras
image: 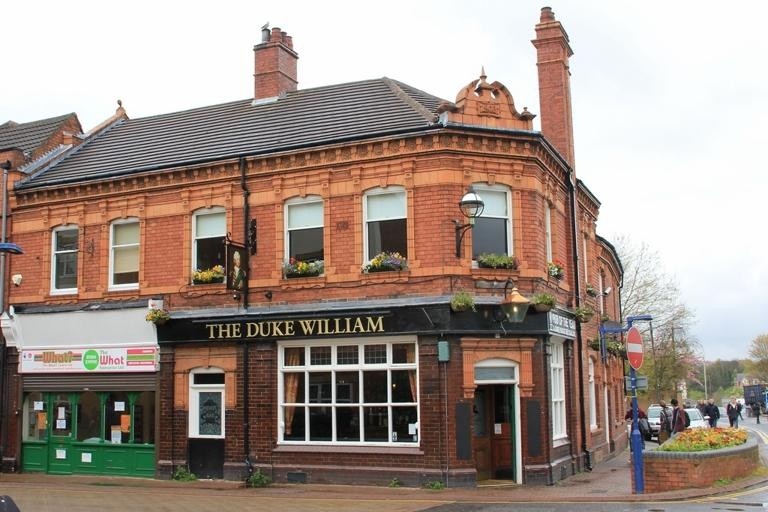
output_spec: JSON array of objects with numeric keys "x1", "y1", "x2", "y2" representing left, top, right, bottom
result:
[{"x1": 604, "y1": 287, "x2": 611, "y2": 295}]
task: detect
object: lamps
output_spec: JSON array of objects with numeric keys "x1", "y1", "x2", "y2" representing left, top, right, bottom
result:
[
  {"x1": 451, "y1": 192, "x2": 484, "y2": 258},
  {"x1": 495, "y1": 278, "x2": 530, "y2": 324}
]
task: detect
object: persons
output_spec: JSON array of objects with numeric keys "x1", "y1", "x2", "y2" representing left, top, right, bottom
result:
[
  {"x1": 228, "y1": 250, "x2": 244, "y2": 289},
  {"x1": 624, "y1": 400, "x2": 647, "y2": 451},
  {"x1": 670, "y1": 398, "x2": 687, "y2": 435},
  {"x1": 696, "y1": 396, "x2": 762, "y2": 429},
  {"x1": 655, "y1": 399, "x2": 673, "y2": 445}
]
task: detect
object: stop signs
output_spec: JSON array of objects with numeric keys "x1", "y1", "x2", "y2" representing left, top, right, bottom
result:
[{"x1": 623, "y1": 326, "x2": 644, "y2": 371}]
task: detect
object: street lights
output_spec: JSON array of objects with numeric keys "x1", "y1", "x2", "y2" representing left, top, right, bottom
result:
[
  {"x1": 598, "y1": 315, "x2": 654, "y2": 496},
  {"x1": 0, "y1": 243, "x2": 23, "y2": 255},
  {"x1": 668, "y1": 322, "x2": 686, "y2": 406}
]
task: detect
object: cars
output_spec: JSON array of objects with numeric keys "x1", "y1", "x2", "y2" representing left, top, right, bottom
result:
[
  {"x1": 679, "y1": 407, "x2": 711, "y2": 431},
  {"x1": 714, "y1": 407, "x2": 729, "y2": 423}
]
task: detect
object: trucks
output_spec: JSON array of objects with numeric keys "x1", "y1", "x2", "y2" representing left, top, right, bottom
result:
[{"x1": 741, "y1": 383, "x2": 767, "y2": 417}]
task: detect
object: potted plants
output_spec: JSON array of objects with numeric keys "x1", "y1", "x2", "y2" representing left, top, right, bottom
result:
[
  {"x1": 533, "y1": 293, "x2": 556, "y2": 313},
  {"x1": 478, "y1": 252, "x2": 522, "y2": 270},
  {"x1": 451, "y1": 292, "x2": 477, "y2": 314},
  {"x1": 576, "y1": 308, "x2": 594, "y2": 322}
]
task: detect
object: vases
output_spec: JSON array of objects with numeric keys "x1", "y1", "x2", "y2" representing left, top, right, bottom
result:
[
  {"x1": 286, "y1": 271, "x2": 319, "y2": 278},
  {"x1": 193, "y1": 278, "x2": 224, "y2": 284},
  {"x1": 153, "y1": 318, "x2": 166, "y2": 325}
]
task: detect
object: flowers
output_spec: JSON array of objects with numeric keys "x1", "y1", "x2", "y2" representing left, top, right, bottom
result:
[
  {"x1": 548, "y1": 263, "x2": 564, "y2": 278},
  {"x1": 282, "y1": 257, "x2": 324, "y2": 274},
  {"x1": 193, "y1": 264, "x2": 224, "y2": 283},
  {"x1": 366, "y1": 251, "x2": 406, "y2": 273},
  {"x1": 146, "y1": 310, "x2": 170, "y2": 322}
]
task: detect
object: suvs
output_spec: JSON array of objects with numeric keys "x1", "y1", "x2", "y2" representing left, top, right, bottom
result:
[{"x1": 641, "y1": 403, "x2": 674, "y2": 442}]
